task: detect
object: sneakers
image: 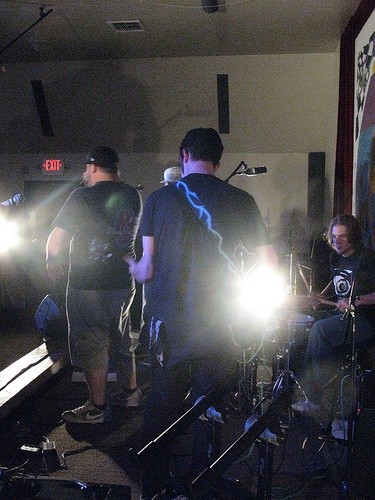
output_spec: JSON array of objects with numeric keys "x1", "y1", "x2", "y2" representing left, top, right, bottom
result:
[
  {"x1": 106, "y1": 390, "x2": 142, "y2": 409},
  {"x1": 60, "y1": 404, "x2": 104, "y2": 427}
]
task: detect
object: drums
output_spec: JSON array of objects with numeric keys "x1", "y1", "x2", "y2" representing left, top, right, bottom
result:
[
  {"x1": 277, "y1": 296, "x2": 322, "y2": 321},
  {"x1": 252, "y1": 312, "x2": 317, "y2": 351}
]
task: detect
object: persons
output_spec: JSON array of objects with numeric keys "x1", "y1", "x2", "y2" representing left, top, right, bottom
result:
[
  {"x1": 292, "y1": 214, "x2": 375, "y2": 442},
  {"x1": 160, "y1": 167, "x2": 182, "y2": 186},
  {"x1": 125, "y1": 127, "x2": 276, "y2": 500},
  {"x1": 47, "y1": 147, "x2": 142, "y2": 424}
]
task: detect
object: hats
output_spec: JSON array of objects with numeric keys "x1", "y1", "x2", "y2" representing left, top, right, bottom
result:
[
  {"x1": 159, "y1": 164, "x2": 183, "y2": 185},
  {"x1": 75, "y1": 146, "x2": 120, "y2": 166}
]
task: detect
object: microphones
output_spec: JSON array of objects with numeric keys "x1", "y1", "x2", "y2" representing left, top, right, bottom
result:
[
  {"x1": 239, "y1": 167, "x2": 267, "y2": 176},
  {"x1": 0, "y1": 194, "x2": 25, "y2": 208}
]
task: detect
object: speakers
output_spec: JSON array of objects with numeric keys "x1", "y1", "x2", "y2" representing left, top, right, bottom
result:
[{"x1": 35, "y1": 293, "x2": 66, "y2": 334}]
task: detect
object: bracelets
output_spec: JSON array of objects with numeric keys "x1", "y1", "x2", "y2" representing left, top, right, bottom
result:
[{"x1": 45, "y1": 256, "x2": 59, "y2": 262}]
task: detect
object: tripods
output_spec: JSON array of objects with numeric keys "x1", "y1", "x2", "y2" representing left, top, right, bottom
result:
[{"x1": 272, "y1": 324, "x2": 309, "y2": 403}]
